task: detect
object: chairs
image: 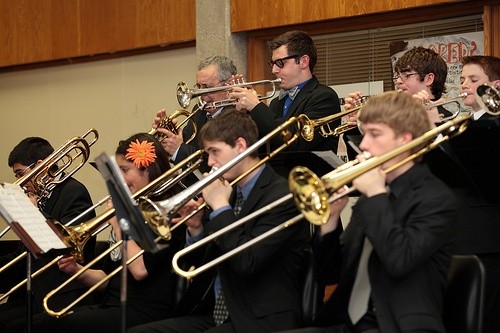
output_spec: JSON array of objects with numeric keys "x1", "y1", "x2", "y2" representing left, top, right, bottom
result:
[
  {"x1": 299, "y1": 241, "x2": 325, "y2": 328},
  {"x1": 447, "y1": 255, "x2": 485, "y2": 333}
]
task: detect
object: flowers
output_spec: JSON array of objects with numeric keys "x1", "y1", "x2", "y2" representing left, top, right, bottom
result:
[{"x1": 125, "y1": 139, "x2": 157, "y2": 168}]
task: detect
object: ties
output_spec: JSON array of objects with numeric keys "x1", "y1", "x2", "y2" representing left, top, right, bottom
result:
[
  {"x1": 214, "y1": 289, "x2": 229, "y2": 326},
  {"x1": 347, "y1": 237, "x2": 373, "y2": 324}
]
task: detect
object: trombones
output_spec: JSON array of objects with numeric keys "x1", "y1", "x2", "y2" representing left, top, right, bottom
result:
[
  {"x1": 0, "y1": 147, "x2": 206, "y2": 303},
  {"x1": 0, "y1": 127, "x2": 100, "y2": 239},
  {"x1": 42, "y1": 114, "x2": 304, "y2": 318},
  {"x1": 171, "y1": 107, "x2": 473, "y2": 280}
]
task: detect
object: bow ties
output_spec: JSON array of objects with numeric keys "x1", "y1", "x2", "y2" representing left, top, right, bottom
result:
[{"x1": 279, "y1": 85, "x2": 300, "y2": 100}]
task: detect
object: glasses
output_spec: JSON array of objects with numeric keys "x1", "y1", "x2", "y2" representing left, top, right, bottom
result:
[
  {"x1": 15, "y1": 163, "x2": 35, "y2": 179},
  {"x1": 267, "y1": 55, "x2": 303, "y2": 68},
  {"x1": 393, "y1": 72, "x2": 424, "y2": 82},
  {"x1": 194, "y1": 80, "x2": 227, "y2": 89}
]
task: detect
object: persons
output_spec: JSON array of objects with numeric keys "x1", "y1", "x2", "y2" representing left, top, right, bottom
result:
[
  {"x1": 57, "y1": 133, "x2": 172, "y2": 333},
  {"x1": 127, "y1": 110, "x2": 306, "y2": 333},
  {"x1": 345, "y1": 47, "x2": 460, "y2": 186},
  {"x1": 0, "y1": 137, "x2": 97, "y2": 304},
  {"x1": 311, "y1": 90, "x2": 456, "y2": 333},
  {"x1": 150, "y1": 55, "x2": 268, "y2": 174},
  {"x1": 450, "y1": 55, "x2": 500, "y2": 255},
  {"x1": 226, "y1": 30, "x2": 342, "y2": 177}
]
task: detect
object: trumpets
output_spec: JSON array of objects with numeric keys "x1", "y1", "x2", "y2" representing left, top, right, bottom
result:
[
  {"x1": 355, "y1": 91, "x2": 470, "y2": 136},
  {"x1": 175, "y1": 77, "x2": 282, "y2": 109},
  {"x1": 113, "y1": 93, "x2": 209, "y2": 162},
  {"x1": 474, "y1": 81, "x2": 500, "y2": 116},
  {"x1": 295, "y1": 88, "x2": 405, "y2": 142}
]
